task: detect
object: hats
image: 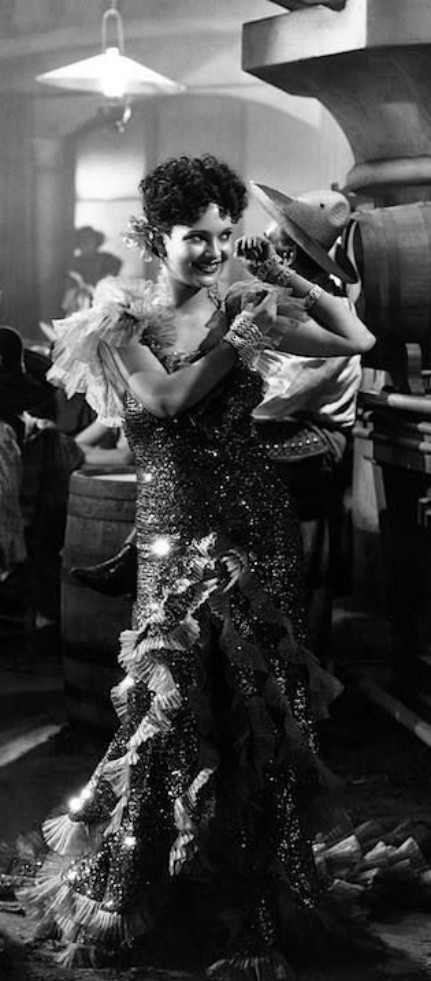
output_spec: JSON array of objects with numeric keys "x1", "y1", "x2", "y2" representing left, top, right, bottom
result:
[
  {"x1": 248, "y1": 177, "x2": 360, "y2": 288},
  {"x1": 75, "y1": 227, "x2": 105, "y2": 249}
]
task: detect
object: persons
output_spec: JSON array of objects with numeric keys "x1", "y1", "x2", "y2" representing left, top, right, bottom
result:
[
  {"x1": 241, "y1": 178, "x2": 362, "y2": 599},
  {"x1": 0, "y1": 225, "x2": 120, "y2": 702},
  {"x1": 0, "y1": 156, "x2": 408, "y2": 980}
]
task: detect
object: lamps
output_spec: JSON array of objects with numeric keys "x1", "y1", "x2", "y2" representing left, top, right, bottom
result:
[{"x1": 34, "y1": 0, "x2": 185, "y2": 136}]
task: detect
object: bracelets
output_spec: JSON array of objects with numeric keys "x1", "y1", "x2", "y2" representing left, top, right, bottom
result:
[
  {"x1": 226, "y1": 310, "x2": 264, "y2": 365},
  {"x1": 257, "y1": 253, "x2": 324, "y2": 309}
]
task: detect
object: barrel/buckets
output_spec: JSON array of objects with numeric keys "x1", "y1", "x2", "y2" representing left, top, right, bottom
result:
[
  {"x1": 341, "y1": 202, "x2": 431, "y2": 342},
  {"x1": 60, "y1": 465, "x2": 141, "y2": 736}
]
